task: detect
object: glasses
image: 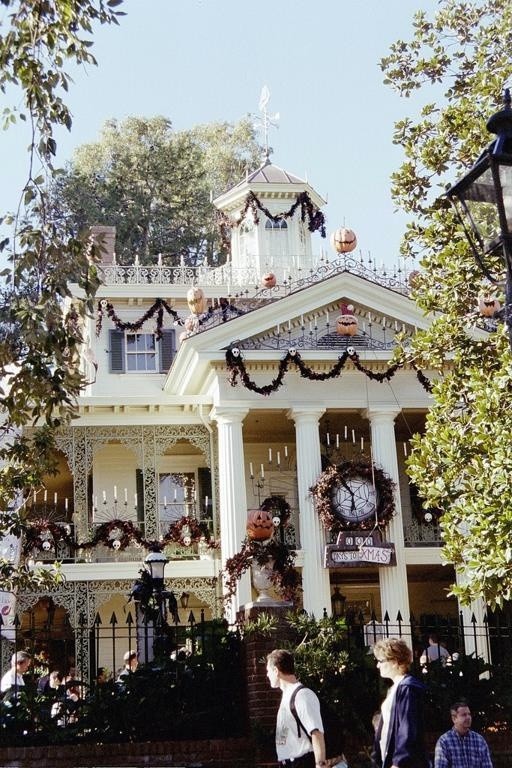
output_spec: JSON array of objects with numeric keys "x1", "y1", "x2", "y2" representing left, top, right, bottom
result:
[{"x1": 377, "y1": 658, "x2": 393, "y2": 663}]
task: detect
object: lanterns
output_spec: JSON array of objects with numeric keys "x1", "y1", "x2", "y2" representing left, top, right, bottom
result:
[
  {"x1": 476, "y1": 290, "x2": 500, "y2": 317},
  {"x1": 186, "y1": 288, "x2": 204, "y2": 315},
  {"x1": 335, "y1": 315, "x2": 358, "y2": 336},
  {"x1": 247, "y1": 509, "x2": 272, "y2": 542},
  {"x1": 262, "y1": 272, "x2": 276, "y2": 289},
  {"x1": 409, "y1": 271, "x2": 421, "y2": 289},
  {"x1": 331, "y1": 227, "x2": 358, "y2": 255}
]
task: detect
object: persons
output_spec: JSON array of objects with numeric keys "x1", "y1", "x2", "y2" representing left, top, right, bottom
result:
[
  {"x1": 266, "y1": 649, "x2": 326, "y2": 768},
  {"x1": 1, "y1": 647, "x2": 139, "y2": 729},
  {"x1": 414, "y1": 628, "x2": 450, "y2": 669},
  {"x1": 369, "y1": 638, "x2": 433, "y2": 767},
  {"x1": 434, "y1": 702, "x2": 493, "y2": 768}
]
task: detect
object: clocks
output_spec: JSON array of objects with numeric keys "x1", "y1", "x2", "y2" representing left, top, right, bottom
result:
[{"x1": 330, "y1": 473, "x2": 380, "y2": 523}]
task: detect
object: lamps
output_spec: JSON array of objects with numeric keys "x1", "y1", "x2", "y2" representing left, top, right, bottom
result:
[
  {"x1": 18, "y1": 490, "x2": 69, "y2": 552},
  {"x1": 268, "y1": 445, "x2": 289, "y2": 470},
  {"x1": 89, "y1": 485, "x2": 138, "y2": 551},
  {"x1": 250, "y1": 462, "x2": 267, "y2": 510},
  {"x1": 402, "y1": 442, "x2": 409, "y2": 459},
  {"x1": 326, "y1": 424, "x2": 364, "y2": 461},
  {"x1": 164, "y1": 487, "x2": 209, "y2": 546}
]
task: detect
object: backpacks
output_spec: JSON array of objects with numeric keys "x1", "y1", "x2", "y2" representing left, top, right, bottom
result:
[{"x1": 290, "y1": 684, "x2": 344, "y2": 759}]
